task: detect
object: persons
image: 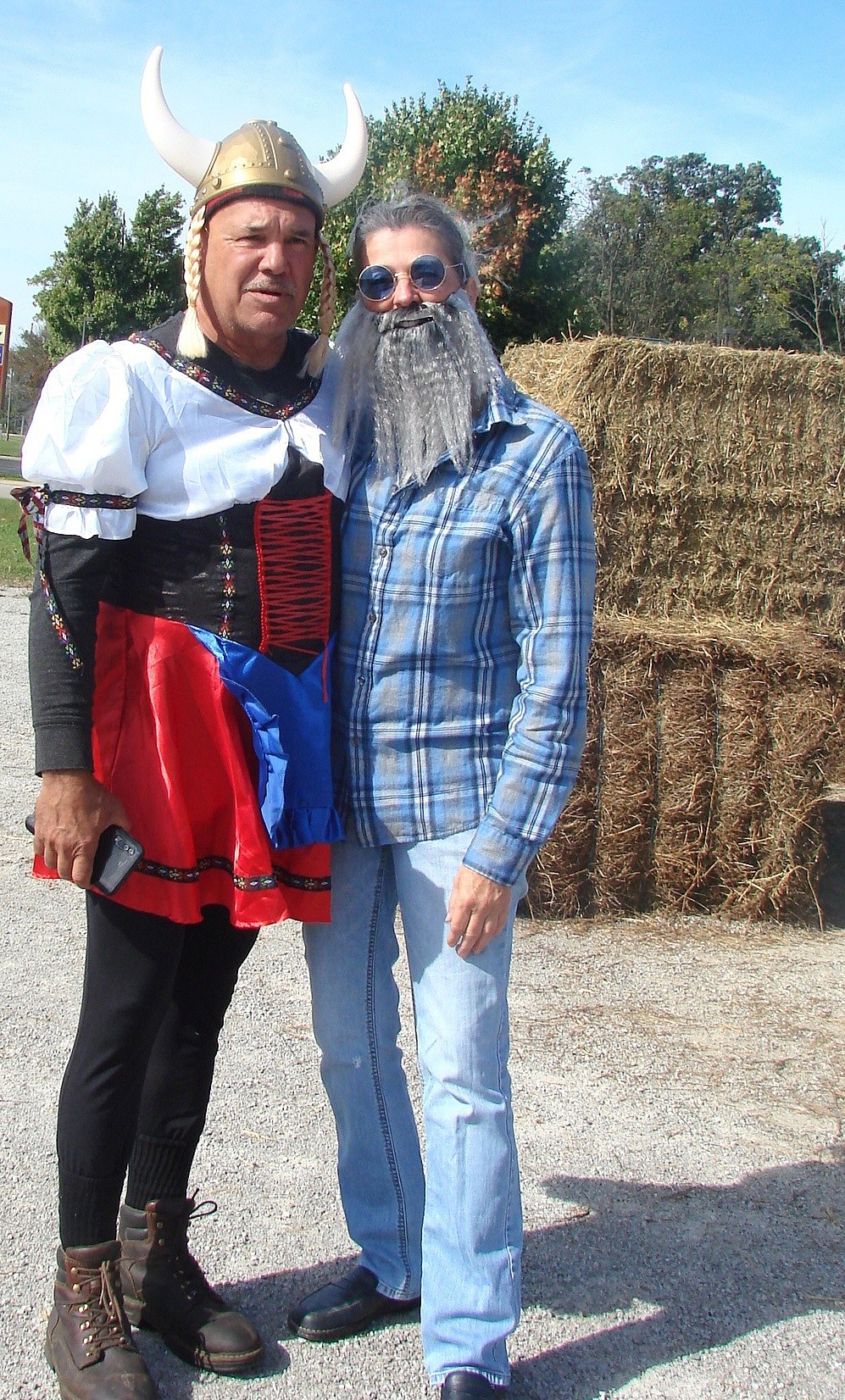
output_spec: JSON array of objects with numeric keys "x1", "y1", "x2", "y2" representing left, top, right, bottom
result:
[
  {"x1": 291, "y1": 191, "x2": 597, "y2": 1400},
  {"x1": 10, "y1": 41, "x2": 368, "y2": 1400}
]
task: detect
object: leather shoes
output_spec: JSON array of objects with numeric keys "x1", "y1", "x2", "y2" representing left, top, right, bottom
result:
[
  {"x1": 288, "y1": 1267, "x2": 399, "y2": 1340},
  {"x1": 441, "y1": 1372, "x2": 506, "y2": 1400}
]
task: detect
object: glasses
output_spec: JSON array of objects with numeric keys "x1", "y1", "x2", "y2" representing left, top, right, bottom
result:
[{"x1": 352, "y1": 255, "x2": 463, "y2": 302}]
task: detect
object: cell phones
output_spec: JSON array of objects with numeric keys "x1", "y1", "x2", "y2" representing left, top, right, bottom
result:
[{"x1": 26, "y1": 810, "x2": 144, "y2": 896}]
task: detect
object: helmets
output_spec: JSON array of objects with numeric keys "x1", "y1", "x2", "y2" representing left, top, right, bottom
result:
[{"x1": 140, "y1": 47, "x2": 371, "y2": 229}]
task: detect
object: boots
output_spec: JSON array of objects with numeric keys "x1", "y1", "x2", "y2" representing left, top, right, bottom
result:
[
  {"x1": 113, "y1": 1189, "x2": 267, "y2": 1372},
  {"x1": 44, "y1": 1232, "x2": 163, "y2": 1400}
]
